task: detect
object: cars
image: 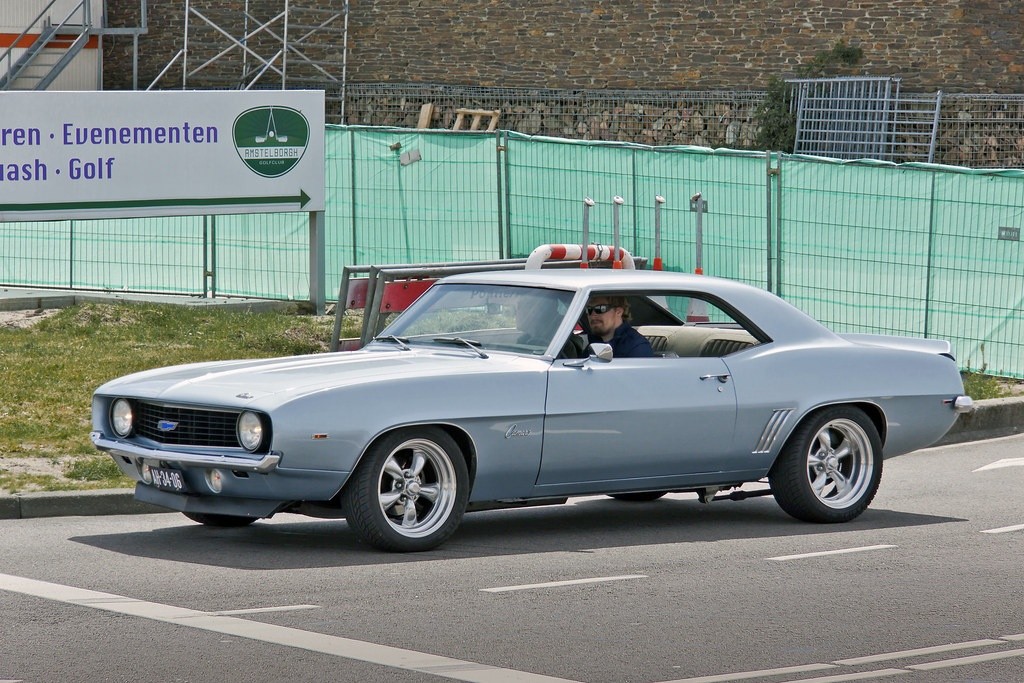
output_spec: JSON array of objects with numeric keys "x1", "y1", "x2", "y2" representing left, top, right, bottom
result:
[{"x1": 87, "y1": 268, "x2": 973, "y2": 555}]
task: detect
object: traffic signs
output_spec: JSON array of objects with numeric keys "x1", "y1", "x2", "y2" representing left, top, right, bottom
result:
[{"x1": 1, "y1": 89, "x2": 326, "y2": 222}]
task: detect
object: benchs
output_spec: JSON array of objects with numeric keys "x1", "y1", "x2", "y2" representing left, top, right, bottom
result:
[{"x1": 631, "y1": 326, "x2": 761, "y2": 357}]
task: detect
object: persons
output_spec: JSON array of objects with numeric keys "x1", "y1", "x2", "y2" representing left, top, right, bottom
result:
[
  {"x1": 583, "y1": 295, "x2": 652, "y2": 358},
  {"x1": 515, "y1": 294, "x2": 582, "y2": 360}
]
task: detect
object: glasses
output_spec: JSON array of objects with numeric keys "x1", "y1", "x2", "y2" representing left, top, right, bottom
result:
[{"x1": 585, "y1": 304, "x2": 622, "y2": 314}]
